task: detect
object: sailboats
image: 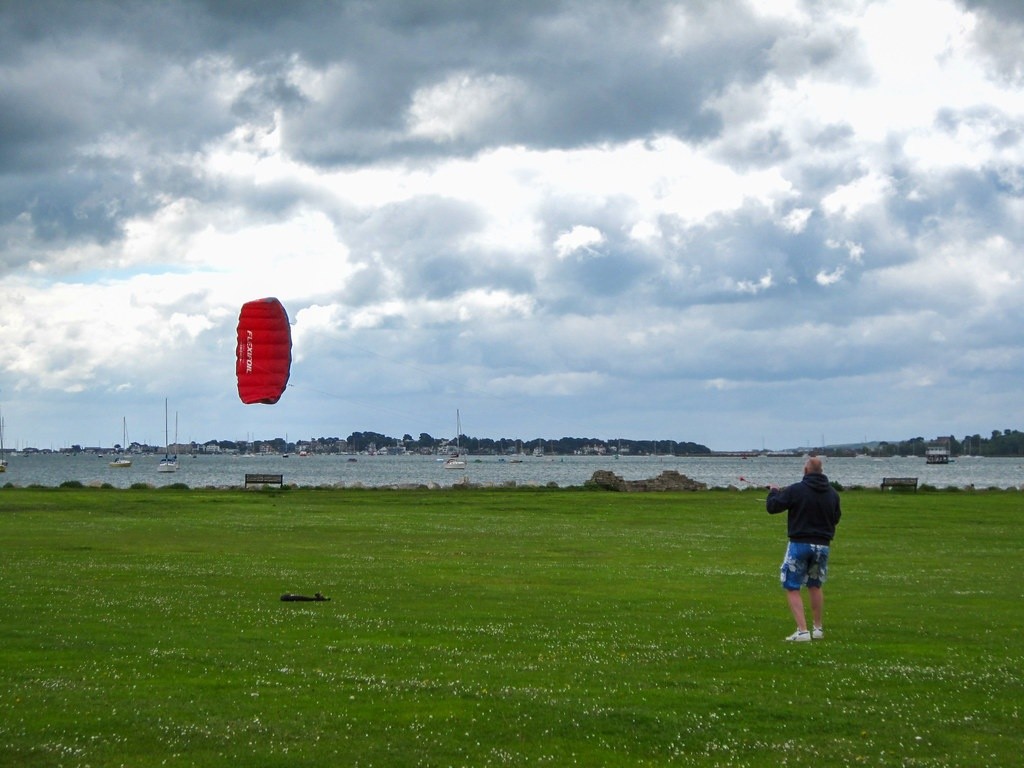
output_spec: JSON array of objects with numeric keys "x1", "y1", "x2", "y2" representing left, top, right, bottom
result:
[
  {"x1": 98, "y1": 441, "x2": 103, "y2": 457},
  {"x1": 110, "y1": 416, "x2": 133, "y2": 468},
  {"x1": 0, "y1": 417, "x2": 8, "y2": 472},
  {"x1": 511, "y1": 436, "x2": 523, "y2": 463},
  {"x1": 283, "y1": 433, "x2": 289, "y2": 458},
  {"x1": 536, "y1": 438, "x2": 542, "y2": 458},
  {"x1": 444, "y1": 408, "x2": 468, "y2": 469},
  {"x1": 157, "y1": 397, "x2": 178, "y2": 472}
]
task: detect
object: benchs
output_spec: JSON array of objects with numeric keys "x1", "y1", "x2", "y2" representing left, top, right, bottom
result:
[
  {"x1": 881, "y1": 477, "x2": 917, "y2": 493},
  {"x1": 245, "y1": 474, "x2": 283, "y2": 489}
]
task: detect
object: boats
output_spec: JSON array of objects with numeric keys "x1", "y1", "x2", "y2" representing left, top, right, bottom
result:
[
  {"x1": 348, "y1": 458, "x2": 357, "y2": 462},
  {"x1": 925, "y1": 447, "x2": 951, "y2": 464}
]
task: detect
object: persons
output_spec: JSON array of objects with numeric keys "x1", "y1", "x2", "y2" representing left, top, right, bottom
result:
[{"x1": 766, "y1": 457, "x2": 842, "y2": 642}]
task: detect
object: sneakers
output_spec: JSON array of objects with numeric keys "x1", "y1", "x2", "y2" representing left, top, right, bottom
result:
[
  {"x1": 786, "y1": 628, "x2": 812, "y2": 641},
  {"x1": 812, "y1": 625, "x2": 824, "y2": 639}
]
